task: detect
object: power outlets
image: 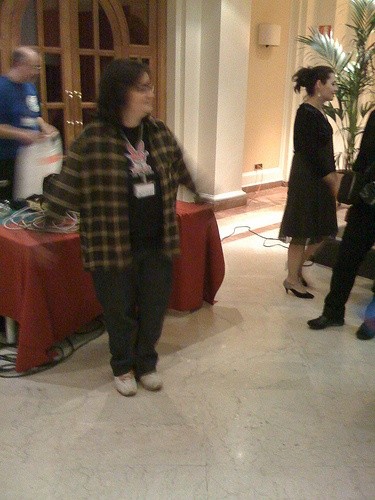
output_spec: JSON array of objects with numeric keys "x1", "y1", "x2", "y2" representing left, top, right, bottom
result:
[{"x1": 254, "y1": 164, "x2": 263, "y2": 168}]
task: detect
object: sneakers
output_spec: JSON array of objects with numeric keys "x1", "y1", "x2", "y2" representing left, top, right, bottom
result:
[
  {"x1": 115, "y1": 369, "x2": 137, "y2": 397},
  {"x1": 139, "y1": 372, "x2": 164, "y2": 391}
]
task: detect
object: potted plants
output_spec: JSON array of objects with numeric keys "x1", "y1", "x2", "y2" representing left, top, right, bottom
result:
[{"x1": 295, "y1": 0, "x2": 375, "y2": 205}]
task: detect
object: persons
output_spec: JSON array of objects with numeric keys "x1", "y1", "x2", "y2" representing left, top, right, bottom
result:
[
  {"x1": 0, "y1": 47, "x2": 199, "y2": 396},
  {"x1": 278, "y1": 66, "x2": 375, "y2": 341}
]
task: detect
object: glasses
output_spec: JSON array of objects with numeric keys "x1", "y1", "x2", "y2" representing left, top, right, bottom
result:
[{"x1": 132, "y1": 81, "x2": 153, "y2": 92}]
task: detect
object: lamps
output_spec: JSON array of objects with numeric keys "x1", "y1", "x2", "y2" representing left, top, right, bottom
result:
[{"x1": 259, "y1": 23, "x2": 281, "y2": 47}]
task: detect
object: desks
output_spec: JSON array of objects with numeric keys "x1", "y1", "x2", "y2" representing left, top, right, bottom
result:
[{"x1": 0, "y1": 199, "x2": 225, "y2": 373}]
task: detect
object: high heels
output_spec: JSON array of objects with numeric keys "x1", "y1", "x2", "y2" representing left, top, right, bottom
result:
[{"x1": 283, "y1": 275, "x2": 314, "y2": 298}]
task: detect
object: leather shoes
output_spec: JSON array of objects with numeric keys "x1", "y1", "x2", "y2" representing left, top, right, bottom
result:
[
  {"x1": 357, "y1": 317, "x2": 375, "y2": 340},
  {"x1": 307, "y1": 310, "x2": 345, "y2": 329}
]
